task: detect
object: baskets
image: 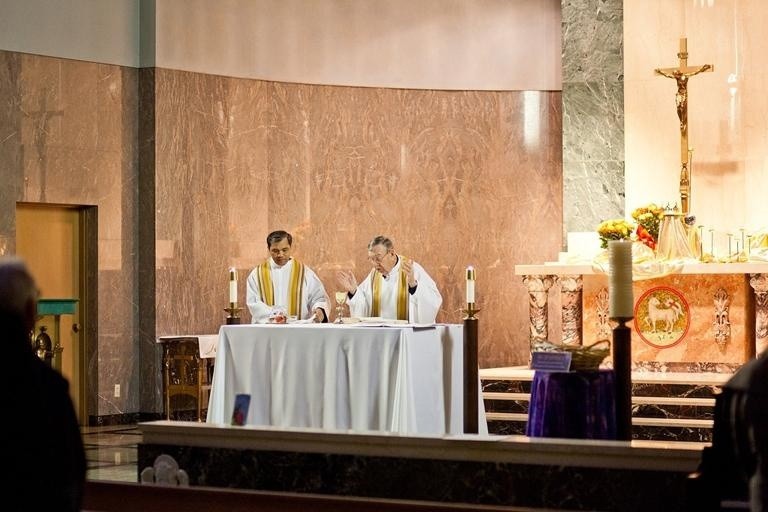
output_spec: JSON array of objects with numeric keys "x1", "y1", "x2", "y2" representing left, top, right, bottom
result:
[{"x1": 533, "y1": 335, "x2": 611, "y2": 371}]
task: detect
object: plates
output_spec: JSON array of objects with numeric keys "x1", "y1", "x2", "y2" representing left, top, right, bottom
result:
[
  {"x1": 290, "y1": 319, "x2": 314, "y2": 324},
  {"x1": 359, "y1": 316, "x2": 408, "y2": 325}
]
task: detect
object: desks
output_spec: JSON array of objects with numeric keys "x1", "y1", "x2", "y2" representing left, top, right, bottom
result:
[
  {"x1": 206, "y1": 322, "x2": 490, "y2": 436},
  {"x1": 156, "y1": 335, "x2": 219, "y2": 421}
]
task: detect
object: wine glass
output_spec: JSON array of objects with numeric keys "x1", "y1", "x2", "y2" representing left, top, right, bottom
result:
[{"x1": 335, "y1": 291, "x2": 346, "y2": 324}]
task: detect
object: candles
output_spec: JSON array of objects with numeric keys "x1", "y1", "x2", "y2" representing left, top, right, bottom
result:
[
  {"x1": 610, "y1": 239, "x2": 633, "y2": 317},
  {"x1": 466, "y1": 266, "x2": 475, "y2": 304},
  {"x1": 229, "y1": 269, "x2": 238, "y2": 307}
]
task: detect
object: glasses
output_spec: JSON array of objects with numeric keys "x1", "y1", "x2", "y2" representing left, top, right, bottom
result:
[{"x1": 367, "y1": 252, "x2": 389, "y2": 262}]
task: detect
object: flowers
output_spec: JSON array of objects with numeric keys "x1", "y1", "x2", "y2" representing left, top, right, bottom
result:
[
  {"x1": 597, "y1": 219, "x2": 634, "y2": 249},
  {"x1": 631, "y1": 204, "x2": 665, "y2": 240}
]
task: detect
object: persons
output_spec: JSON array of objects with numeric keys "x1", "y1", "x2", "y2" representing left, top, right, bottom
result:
[
  {"x1": 245, "y1": 230, "x2": 333, "y2": 326},
  {"x1": 0, "y1": 260, "x2": 91, "y2": 512},
  {"x1": 335, "y1": 236, "x2": 444, "y2": 325},
  {"x1": 653, "y1": 64, "x2": 712, "y2": 138}
]
